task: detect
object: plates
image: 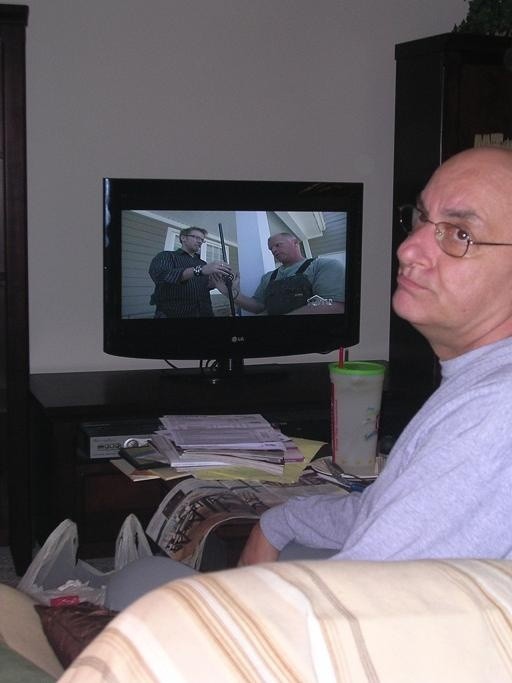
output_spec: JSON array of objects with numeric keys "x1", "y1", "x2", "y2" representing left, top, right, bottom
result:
[{"x1": 310, "y1": 455, "x2": 386, "y2": 479}]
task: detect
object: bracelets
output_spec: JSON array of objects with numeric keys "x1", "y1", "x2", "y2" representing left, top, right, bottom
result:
[
  {"x1": 193, "y1": 264, "x2": 202, "y2": 277},
  {"x1": 231, "y1": 287, "x2": 239, "y2": 300}
]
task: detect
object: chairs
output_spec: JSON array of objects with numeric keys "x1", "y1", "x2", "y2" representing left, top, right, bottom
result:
[{"x1": 1, "y1": 557, "x2": 512, "y2": 676}]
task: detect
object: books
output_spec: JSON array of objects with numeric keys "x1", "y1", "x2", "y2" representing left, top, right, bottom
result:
[{"x1": 110, "y1": 414, "x2": 350, "y2": 570}]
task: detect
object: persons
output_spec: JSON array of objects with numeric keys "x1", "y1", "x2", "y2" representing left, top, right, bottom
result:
[
  {"x1": 239, "y1": 146, "x2": 512, "y2": 566},
  {"x1": 149, "y1": 227, "x2": 232, "y2": 319},
  {"x1": 211, "y1": 232, "x2": 345, "y2": 317}
]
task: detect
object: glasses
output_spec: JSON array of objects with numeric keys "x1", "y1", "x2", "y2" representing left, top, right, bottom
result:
[
  {"x1": 399, "y1": 205, "x2": 512, "y2": 259},
  {"x1": 184, "y1": 234, "x2": 206, "y2": 243}
]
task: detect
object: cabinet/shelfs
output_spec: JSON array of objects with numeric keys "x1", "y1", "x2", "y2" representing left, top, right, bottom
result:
[
  {"x1": 11, "y1": 360, "x2": 398, "y2": 573},
  {"x1": 378, "y1": 32, "x2": 510, "y2": 453}
]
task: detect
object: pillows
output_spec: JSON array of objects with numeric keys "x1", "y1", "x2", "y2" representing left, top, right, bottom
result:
[{"x1": 35, "y1": 601, "x2": 121, "y2": 670}]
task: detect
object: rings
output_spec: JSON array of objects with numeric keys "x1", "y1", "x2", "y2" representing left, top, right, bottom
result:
[{"x1": 221, "y1": 264, "x2": 224, "y2": 267}]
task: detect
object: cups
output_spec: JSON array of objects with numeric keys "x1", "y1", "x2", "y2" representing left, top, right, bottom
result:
[{"x1": 327, "y1": 360, "x2": 385, "y2": 475}]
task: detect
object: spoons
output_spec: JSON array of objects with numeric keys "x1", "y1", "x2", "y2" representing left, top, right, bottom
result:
[{"x1": 323, "y1": 458, "x2": 371, "y2": 488}]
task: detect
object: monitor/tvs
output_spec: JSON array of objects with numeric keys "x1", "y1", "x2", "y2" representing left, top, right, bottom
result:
[{"x1": 101, "y1": 176, "x2": 364, "y2": 387}]
